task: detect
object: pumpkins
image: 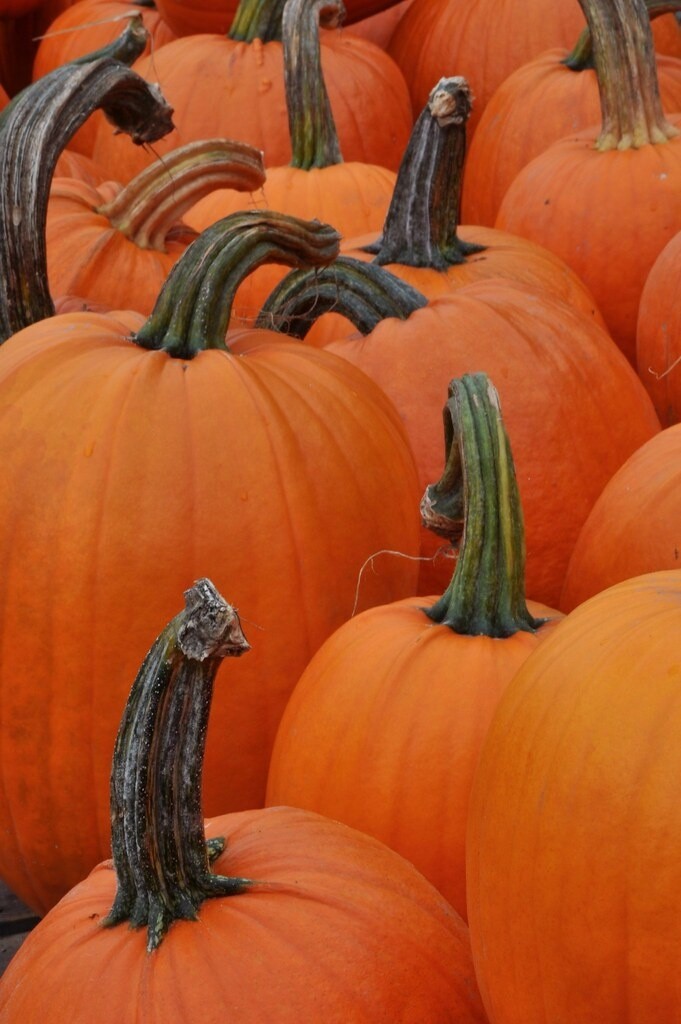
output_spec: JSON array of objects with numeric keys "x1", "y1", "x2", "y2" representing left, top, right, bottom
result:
[{"x1": 0, "y1": 0, "x2": 681, "y2": 1024}]
